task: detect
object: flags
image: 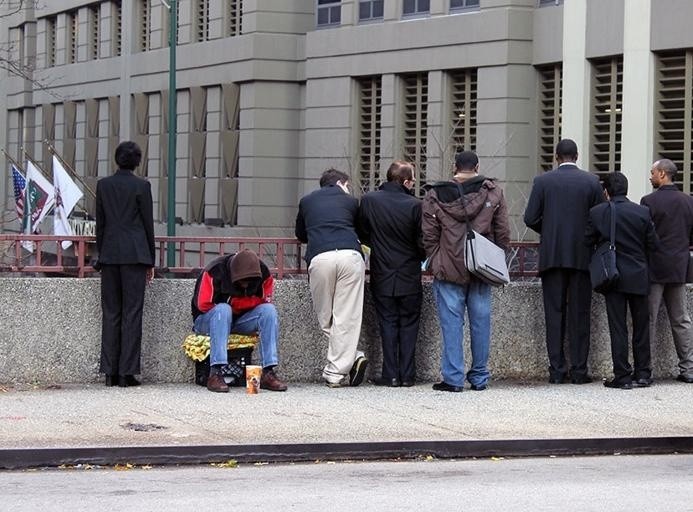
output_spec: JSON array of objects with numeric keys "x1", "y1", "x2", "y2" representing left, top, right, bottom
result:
[
  {"x1": 18, "y1": 159, "x2": 54, "y2": 254},
  {"x1": 10, "y1": 162, "x2": 41, "y2": 234},
  {"x1": 52, "y1": 153, "x2": 84, "y2": 250}
]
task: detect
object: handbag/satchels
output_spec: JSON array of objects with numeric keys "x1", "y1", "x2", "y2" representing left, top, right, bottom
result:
[
  {"x1": 462, "y1": 229, "x2": 511, "y2": 287},
  {"x1": 588, "y1": 239, "x2": 620, "y2": 292}
]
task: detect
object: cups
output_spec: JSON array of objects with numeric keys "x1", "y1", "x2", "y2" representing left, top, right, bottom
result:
[{"x1": 245, "y1": 364, "x2": 262, "y2": 395}]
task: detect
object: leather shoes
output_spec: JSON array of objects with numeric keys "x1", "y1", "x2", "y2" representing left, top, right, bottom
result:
[
  {"x1": 603, "y1": 376, "x2": 634, "y2": 390},
  {"x1": 548, "y1": 376, "x2": 569, "y2": 384},
  {"x1": 259, "y1": 372, "x2": 289, "y2": 392},
  {"x1": 206, "y1": 374, "x2": 230, "y2": 392},
  {"x1": 672, "y1": 375, "x2": 693, "y2": 384},
  {"x1": 470, "y1": 384, "x2": 487, "y2": 391},
  {"x1": 571, "y1": 375, "x2": 595, "y2": 385},
  {"x1": 373, "y1": 377, "x2": 399, "y2": 387},
  {"x1": 432, "y1": 381, "x2": 464, "y2": 392},
  {"x1": 638, "y1": 376, "x2": 654, "y2": 387},
  {"x1": 400, "y1": 381, "x2": 417, "y2": 386}
]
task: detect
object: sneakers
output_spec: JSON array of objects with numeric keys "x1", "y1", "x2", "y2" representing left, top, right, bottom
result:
[
  {"x1": 325, "y1": 379, "x2": 342, "y2": 388},
  {"x1": 349, "y1": 357, "x2": 369, "y2": 386}
]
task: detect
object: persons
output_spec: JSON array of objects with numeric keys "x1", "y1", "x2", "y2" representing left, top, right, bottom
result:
[
  {"x1": 523, "y1": 139, "x2": 605, "y2": 384},
  {"x1": 639, "y1": 158, "x2": 693, "y2": 384},
  {"x1": 420, "y1": 150, "x2": 511, "y2": 392},
  {"x1": 355, "y1": 160, "x2": 426, "y2": 387},
  {"x1": 190, "y1": 248, "x2": 287, "y2": 394},
  {"x1": 589, "y1": 171, "x2": 661, "y2": 389},
  {"x1": 94, "y1": 140, "x2": 157, "y2": 388},
  {"x1": 293, "y1": 168, "x2": 370, "y2": 388}
]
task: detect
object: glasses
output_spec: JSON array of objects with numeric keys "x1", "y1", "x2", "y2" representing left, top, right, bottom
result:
[{"x1": 408, "y1": 178, "x2": 417, "y2": 183}]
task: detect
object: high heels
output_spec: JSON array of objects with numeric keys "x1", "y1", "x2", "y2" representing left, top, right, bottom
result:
[
  {"x1": 104, "y1": 374, "x2": 119, "y2": 386},
  {"x1": 119, "y1": 374, "x2": 142, "y2": 387}
]
